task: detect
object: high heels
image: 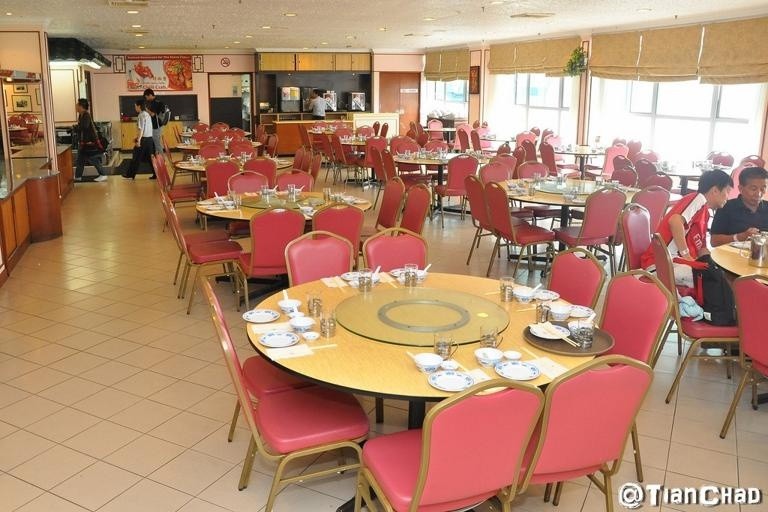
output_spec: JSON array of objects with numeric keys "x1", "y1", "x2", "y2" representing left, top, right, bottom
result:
[
  {"x1": 122, "y1": 173, "x2": 135, "y2": 180},
  {"x1": 149, "y1": 175, "x2": 156, "y2": 178}
]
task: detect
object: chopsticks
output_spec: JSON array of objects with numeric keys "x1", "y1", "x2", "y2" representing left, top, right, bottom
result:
[
  {"x1": 308, "y1": 343, "x2": 337, "y2": 351},
  {"x1": 484, "y1": 291, "x2": 501, "y2": 296},
  {"x1": 331, "y1": 277, "x2": 346, "y2": 293},
  {"x1": 519, "y1": 345, "x2": 538, "y2": 359}
]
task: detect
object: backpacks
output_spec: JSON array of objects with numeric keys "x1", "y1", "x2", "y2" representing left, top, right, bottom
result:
[{"x1": 153, "y1": 99, "x2": 171, "y2": 126}]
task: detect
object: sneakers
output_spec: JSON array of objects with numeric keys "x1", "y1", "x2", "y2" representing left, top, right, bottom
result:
[
  {"x1": 74, "y1": 176, "x2": 81, "y2": 181},
  {"x1": 94, "y1": 175, "x2": 107, "y2": 182}
]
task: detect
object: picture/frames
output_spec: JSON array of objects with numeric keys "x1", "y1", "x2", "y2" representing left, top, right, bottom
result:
[
  {"x1": 11, "y1": 95, "x2": 32, "y2": 112},
  {"x1": 469, "y1": 66, "x2": 479, "y2": 94},
  {"x1": 13, "y1": 84, "x2": 28, "y2": 93},
  {"x1": 35, "y1": 88, "x2": 42, "y2": 105}
]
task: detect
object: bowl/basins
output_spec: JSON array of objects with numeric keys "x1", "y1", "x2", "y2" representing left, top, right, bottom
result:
[
  {"x1": 474, "y1": 348, "x2": 504, "y2": 368},
  {"x1": 415, "y1": 352, "x2": 442, "y2": 374},
  {"x1": 279, "y1": 299, "x2": 302, "y2": 313},
  {"x1": 289, "y1": 311, "x2": 304, "y2": 318},
  {"x1": 503, "y1": 351, "x2": 521, "y2": 362},
  {"x1": 289, "y1": 316, "x2": 316, "y2": 333},
  {"x1": 122, "y1": 116, "x2": 137, "y2": 122},
  {"x1": 440, "y1": 360, "x2": 459, "y2": 371},
  {"x1": 303, "y1": 332, "x2": 320, "y2": 342}
]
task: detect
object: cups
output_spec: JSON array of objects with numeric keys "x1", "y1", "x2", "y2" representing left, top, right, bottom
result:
[
  {"x1": 479, "y1": 325, "x2": 503, "y2": 349},
  {"x1": 185, "y1": 151, "x2": 282, "y2": 172},
  {"x1": 555, "y1": 136, "x2": 604, "y2": 153},
  {"x1": 505, "y1": 174, "x2": 628, "y2": 204},
  {"x1": 309, "y1": 124, "x2": 375, "y2": 148},
  {"x1": 196, "y1": 183, "x2": 354, "y2": 218},
  {"x1": 306, "y1": 290, "x2": 321, "y2": 315},
  {"x1": 180, "y1": 126, "x2": 202, "y2": 144},
  {"x1": 739, "y1": 230, "x2": 768, "y2": 267},
  {"x1": 174, "y1": 116, "x2": 179, "y2": 120},
  {"x1": 658, "y1": 155, "x2": 732, "y2": 173},
  {"x1": 320, "y1": 310, "x2": 337, "y2": 337},
  {"x1": 391, "y1": 149, "x2": 501, "y2": 163},
  {"x1": 500, "y1": 277, "x2": 515, "y2": 302},
  {"x1": 434, "y1": 335, "x2": 459, "y2": 360}
]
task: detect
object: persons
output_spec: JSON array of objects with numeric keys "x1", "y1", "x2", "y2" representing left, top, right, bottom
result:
[
  {"x1": 308, "y1": 89, "x2": 327, "y2": 119},
  {"x1": 70, "y1": 98, "x2": 108, "y2": 181},
  {"x1": 641, "y1": 170, "x2": 734, "y2": 288},
  {"x1": 709, "y1": 166, "x2": 768, "y2": 247},
  {"x1": 141, "y1": 88, "x2": 165, "y2": 153},
  {"x1": 122, "y1": 100, "x2": 157, "y2": 180}
]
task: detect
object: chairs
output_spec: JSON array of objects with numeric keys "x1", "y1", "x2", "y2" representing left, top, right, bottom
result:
[{"x1": 151, "y1": 111, "x2": 768, "y2": 512}]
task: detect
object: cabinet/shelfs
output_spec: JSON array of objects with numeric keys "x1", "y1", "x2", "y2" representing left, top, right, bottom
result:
[
  {"x1": 335, "y1": 53, "x2": 372, "y2": 71},
  {"x1": 120, "y1": 120, "x2": 138, "y2": 153},
  {"x1": 256, "y1": 53, "x2": 336, "y2": 72}
]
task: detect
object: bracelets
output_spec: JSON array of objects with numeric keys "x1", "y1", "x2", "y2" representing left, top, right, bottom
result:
[
  {"x1": 679, "y1": 248, "x2": 689, "y2": 256},
  {"x1": 733, "y1": 234, "x2": 739, "y2": 242}
]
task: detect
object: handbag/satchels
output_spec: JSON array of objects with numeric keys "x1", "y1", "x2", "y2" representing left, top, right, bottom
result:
[
  {"x1": 77, "y1": 137, "x2": 108, "y2": 156},
  {"x1": 674, "y1": 255, "x2": 737, "y2": 327}
]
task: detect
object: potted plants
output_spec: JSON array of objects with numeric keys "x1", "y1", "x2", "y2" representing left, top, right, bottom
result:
[{"x1": 560, "y1": 46, "x2": 590, "y2": 78}]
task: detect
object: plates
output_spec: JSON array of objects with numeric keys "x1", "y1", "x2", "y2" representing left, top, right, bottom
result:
[
  {"x1": 258, "y1": 330, "x2": 300, "y2": 348},
  {"x1": 512, "y1": 282, "x2": 598, "y2": 349},
  {"x1": 341, "y1": 263, "x2": 432, "y2": 294},
  {"x1": 495, "y1": 360, "x2": 541, "y2": 381},
  {"x1": 243, "y1": 309, "x2": 280, "y2": 323},
  {"x1": 427, "y1": 370, "x2": 474, "y2": 392}
]
